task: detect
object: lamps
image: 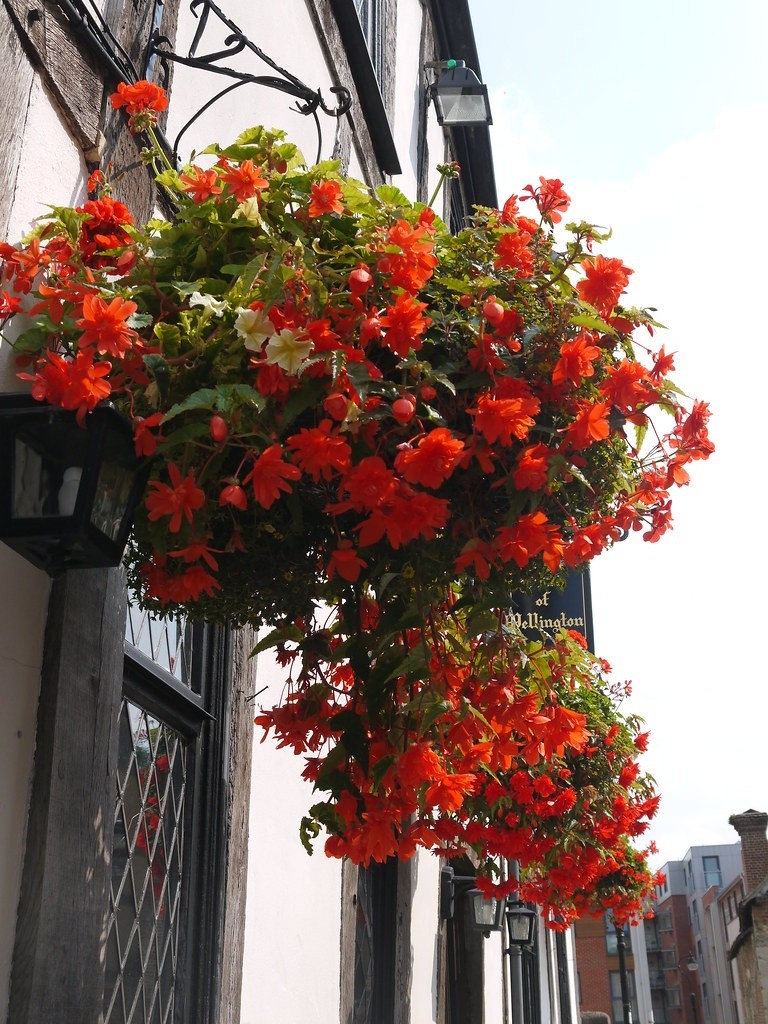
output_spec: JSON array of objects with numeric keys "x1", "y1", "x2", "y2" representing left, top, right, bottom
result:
[
  {"x1": 504, "y1": 899, "x2": 537, "y2": 946},
  {"x1": 421, "y1": 58, "x2": 495, "y2": 128}
]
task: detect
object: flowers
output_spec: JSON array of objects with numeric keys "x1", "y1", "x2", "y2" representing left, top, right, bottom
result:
[{"x1": 1, "y1": 79, "x2": 716, "y2": 934}]
